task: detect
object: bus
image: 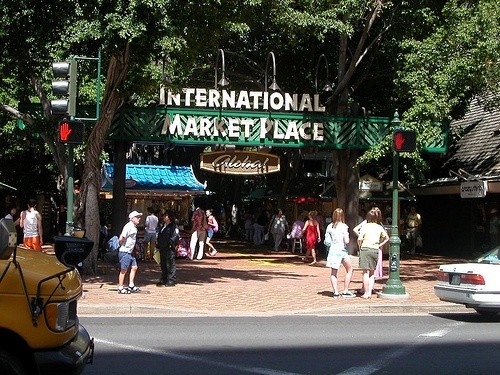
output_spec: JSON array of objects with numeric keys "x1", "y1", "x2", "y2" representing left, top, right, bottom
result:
[{"x1": 0, "y1": 218, "x2": 94, "y2": 375}]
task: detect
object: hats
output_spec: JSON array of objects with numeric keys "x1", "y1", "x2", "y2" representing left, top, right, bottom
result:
[
  {"x1": 129, "y1": 211, "x2": 143, "y2": 218},
  {"x1": 162, "y1": 209, "x2": 174, "y2": 217}
]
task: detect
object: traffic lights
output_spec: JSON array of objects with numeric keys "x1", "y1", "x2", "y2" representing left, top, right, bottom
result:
[
  {"x1": 58, "y1": 121, "x2": 86, "y2": 144},
  {"x1": 50, "y1": 59, "x2": 76, "y2": 116},
  {"x1": 392, "y1": 129, "x2": 416, "y2": 152}
]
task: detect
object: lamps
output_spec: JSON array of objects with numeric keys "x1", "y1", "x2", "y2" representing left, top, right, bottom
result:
[
  {"x1": 215, "y1": 49, "x2": 229, "y2": 89},
  {"x1": 162, "y1": 54, "x2": 172, "y2": 85},
  {"x1": 265, "y1": 52, "x2": 280, "y2": 91},
  {"x1": 315, "y1": 54, "x2": 332, "y2": 94}
]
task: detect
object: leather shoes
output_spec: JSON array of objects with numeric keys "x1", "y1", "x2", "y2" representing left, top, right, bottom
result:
[
  {"x1": 165, "y1": 282, "x2": 175, "y2": 287},
  {"x1": 156, "y1": 280, "x2": 166, "y2": 285}
]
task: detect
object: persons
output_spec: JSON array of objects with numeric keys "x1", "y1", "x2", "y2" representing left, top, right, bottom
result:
[
  {"x1": 405, "y1": 205, "x2": 422, "y2": 255},
  {"x1": 324, "y1": 208, "x2": 356, "y2": 299},
  {"x1": 156, "y1": 209, "x2": 179, "y2": 287},
  {"x1": 141, "y1": 207, "x2": 163, "y2": 263},
  {"x1": 118, "y1": 211, "x2": 143, "y2": 294},
  {"x1": 186, "y1": 200, "x2": 217, "y2": 261},
  {"x1": 300, "y1": 210, "x2": 321, "y2": 265},
  {"x1": 353, "y1": 207, "x2": 389, "y2": 298},
  {"x1": 268, "y1": 208, "x2": 290, "y2": 251},
  {"x1": 291, "y1": 211, "x2": 333, "y2": 252},
  {"x1": 5, "y1": 208, "x2": 20, "y2": 226},
  {"x1": 239, "y1": 204, "x2": 268, "y2": 245},
  {"x1": 487, "y1": 209, "x2": 500, "y2": 251},
  {"x1": 52, "y1": 203, "x2": 67, "y2": 236},
  {"x1": 20, "y1": 199, "x2": 43, "y2": 252}
]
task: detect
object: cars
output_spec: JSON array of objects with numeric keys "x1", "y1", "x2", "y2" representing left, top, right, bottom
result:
[{"x1": 434, "y1": 246, "x2": 500, "y2": 317}]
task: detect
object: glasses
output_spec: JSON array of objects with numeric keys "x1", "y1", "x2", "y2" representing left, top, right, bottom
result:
[{"x1": 133, "y1": 217, "x2": 140, "y2": 219}]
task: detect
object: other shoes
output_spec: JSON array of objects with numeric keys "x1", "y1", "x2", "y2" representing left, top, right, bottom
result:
[
  {"x1": 301, "y1": 259, "x2": 306, "y2": 263},
  {"x1": 211, "y1": 250, "x2": 216, "y2": 256},
  {"x1": 308, "y1": 261, "x2": 316, "y2": 266},
  {"x1": 333, "y1": 293, "x2": 342, "y2": 299},
  {"x1": 342, "y1": 292, "x2": 356, "y2": 297},
  {"x1": 186, "y1": 257, "x2": 192, "y2": 261}
]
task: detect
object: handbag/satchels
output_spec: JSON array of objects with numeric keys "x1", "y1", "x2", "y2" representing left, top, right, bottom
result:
[{"x1": 212, "y1": 218, "x2": 218, "y2": 232}]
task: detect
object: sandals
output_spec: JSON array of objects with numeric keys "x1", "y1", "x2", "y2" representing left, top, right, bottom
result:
[
  {"x1": 118, "y1": 288, "x2": 131, "y2": 294},
  {"x1": 126, "y1": 286, "x2": 141, "y2": 293}
]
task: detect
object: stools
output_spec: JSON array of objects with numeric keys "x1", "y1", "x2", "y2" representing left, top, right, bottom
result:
[{"x1": 292, "y1": 238, "x2": 302, "y2": 254}]
